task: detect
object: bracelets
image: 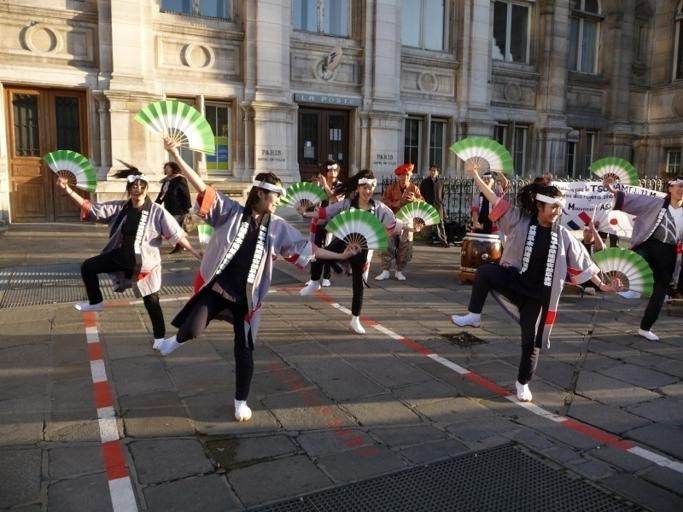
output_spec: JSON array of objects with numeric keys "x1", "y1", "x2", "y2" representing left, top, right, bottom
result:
[{"x1": 69, "y1": 190, "x2": 74, "y2": 196}]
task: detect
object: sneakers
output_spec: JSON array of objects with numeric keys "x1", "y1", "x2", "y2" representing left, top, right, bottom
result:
[
  {"x1": 451, "y1": 314, "x2": 480, "y2": 328},
  {"x1": 515, "y1": 380, "x2": 532, "y2": 402},
  {"x1": 153, "y1": 335, "x2": 185, "y2": 354},
  {"x1": 235, "y1": 404, "x2": 252, "y2": 421},
  {"x1": 300, "y1": 279, "x2": 330, "y2": 294},
  {"x1": 74, "y1": 301, "x2": 103, "y2": 311},
  {"x1": 349, "y1": 321, "x2": 365, "y2": 334},
  {"x1": 375, "y1": 271, "x2": 406, "y2": 281},
  {"x1": 639, "y1": 329, "x2": 659, "y2": 341}
]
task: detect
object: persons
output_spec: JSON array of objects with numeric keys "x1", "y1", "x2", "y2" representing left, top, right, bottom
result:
[
  {"x1": 451, "y1": 161, "x2": 683, "y2": 402},
  {"x1": 156, "y1": 162, "x2": 192, "y2": 255},
  {"x1": 157, "y1": 136, "x2": 361, "y2": 423},
  {"x1": 56, "y1": 173, "x2": 202, "y2": 351},
  {"x1": 300, "y1": 158, "x2": 446, "y2": 337}
]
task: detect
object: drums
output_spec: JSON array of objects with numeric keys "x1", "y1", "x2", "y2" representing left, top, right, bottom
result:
[{"x1": 458, "y1": 232, "x2": 502, "y2": 273}]
